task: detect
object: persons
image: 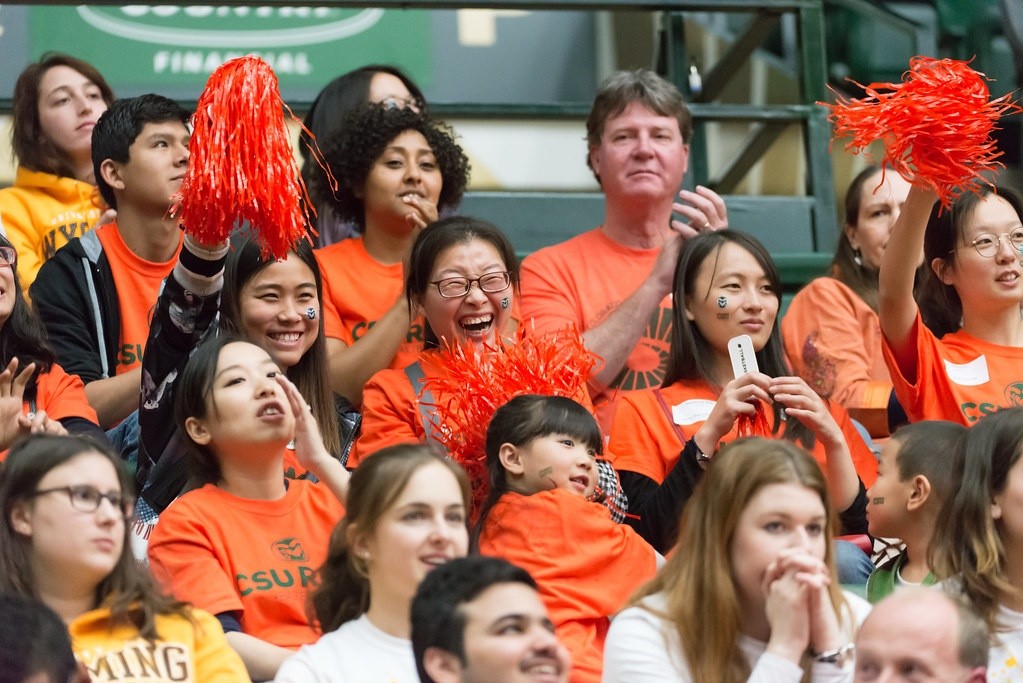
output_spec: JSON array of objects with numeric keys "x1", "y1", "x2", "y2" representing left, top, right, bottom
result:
[
  {"x1": 272, "y1": 445, "x2": 471, "y2": 683},
  {"x1": 518, "y1": 69, "x2": 728, "y2": 449},
  {"x1": 297, "y1": 65, "x2": 424, "y2": 257},
  {"x1": 469, "y1": 394, "x2": 668, "y2": 683},
  {"x1": 779, "y1": 164, "x2": 926, "y2": 448},
  {"x1": 312, "y1": 105, "x2": 471, "y2": 450},
  {"x1": 611, "y1": 227, "x2": 880, "y2": 555},
  {"x1": 409, "y1": 553, "x2": 571, "y2": 683},
  {"x1": 0, "y1": 432, "x2": 251, "y2": 683},
  {"x1": 148, "y1": 339, "x2": 349, "y2": 683},
  {"x1": 599, "y1": 440, "x2": 874, "y2": 683},
  {"x1": 865, "y1": 421, "x2": 969, "y2": 605},
  {"x1": 853, "y1": 590, "x2": 987, "y2": 683},
  {"x1": 880, "y1": 160, "x2": 1023, "y2": 425},
  {"x1": 927, "y1": 405, "x2": 1023, "y2": 683},
  {"x1": 0, "y1": 53, "x2": 340, "y2": 556},
  {"x1": 349, "y1": 217, "x2": 629, "y2": 523}
]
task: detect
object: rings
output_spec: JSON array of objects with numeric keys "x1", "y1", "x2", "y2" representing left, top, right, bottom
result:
[{"x1": 700, "y1": 222, "x2": 710, "y2": 231}]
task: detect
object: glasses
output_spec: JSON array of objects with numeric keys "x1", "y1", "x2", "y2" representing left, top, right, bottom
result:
[
  {"x1": 379, "y1": 95, "x2": 425, "y2": 114},
  {"x1": 0, "y1": 247, "x2": 16, "y2": 267},
  {"x1": 24, "y1": 483, "x2": 135, "y2": 519},
  {"x1": 430, "y1": 271, "x2": 513, "y2": 298},
  {"x1": 949, "y1": 226, "x2": 1023, "y2": 258}
]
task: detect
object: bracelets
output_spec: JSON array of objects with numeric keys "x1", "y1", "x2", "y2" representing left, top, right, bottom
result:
[{"x1": 692, "y1": 434, "x2": 710, "y2": 463}]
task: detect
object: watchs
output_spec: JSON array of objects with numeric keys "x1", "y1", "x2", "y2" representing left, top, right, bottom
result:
[{"x1": 810, "y1": 643, "x2": 854, "y2": 668}]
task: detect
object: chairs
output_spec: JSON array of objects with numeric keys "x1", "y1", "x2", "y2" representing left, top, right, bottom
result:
[
  {"x1": 770, "y1": 252, "x2": 838, "y2": 339},
  {"x1": 703, "y1": 0, "x2": 1023, "y2": 119}
]
